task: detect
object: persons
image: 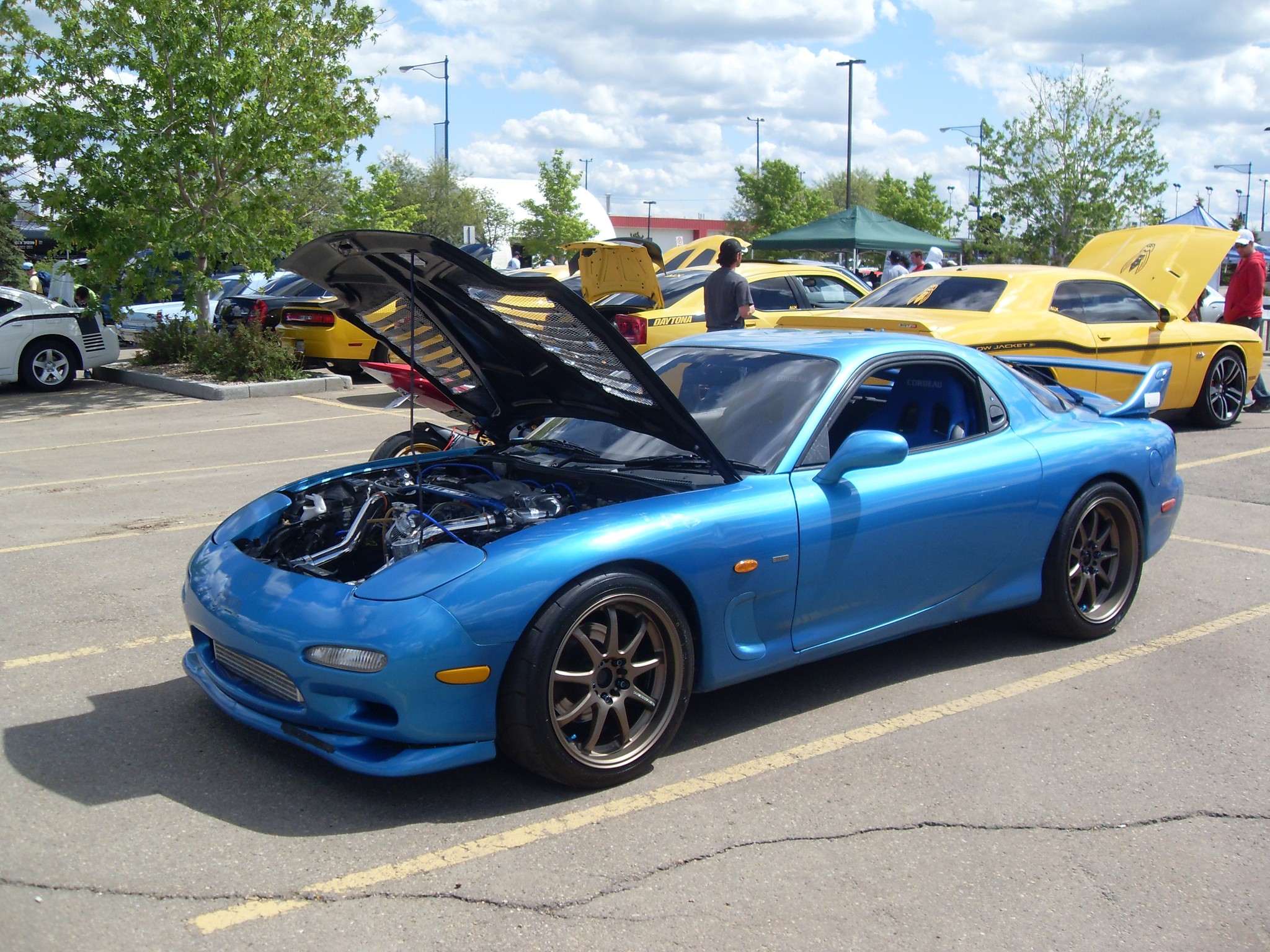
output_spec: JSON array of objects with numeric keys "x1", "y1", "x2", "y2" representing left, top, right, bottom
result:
[
  {"x1": 881, "y1": 250, "x2": 909, "y2": 288},
  {"x1": 910, "y1": 250, "x2": 925, "y2": 272},
  {"x1": 20, "y1": 262, "x2": 52, "y2": 299},
  {"x1": 925, "y1": 246, "x2": 943, "y2": 269},
  {"x1": 1223, "y1": 229, "x2": 1270, "y2": 413},
  {"x1": 73, "y1": 284, "x2": 100, "y2": 378},
  {"x1": 855, "y1": 268, "x2": 863, "y2": 278},
  {"x1": 865, "y1": 271, "x2": 882, "y2": 289},
  {"x1": 1186, "y1": 288, "x2": 1209, "y2": 323},
  {"x1": 702, "y1": 239, "x2": 755, "y2": 333},
  {"x1": 541, "y1": 254, "x2": 555, "y2": 266},
  {"x1": 508, "y1": 251, "x2": 522, "y2": 269}
]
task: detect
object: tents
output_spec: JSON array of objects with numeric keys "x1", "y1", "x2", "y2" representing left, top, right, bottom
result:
[
  {"x1": 1162, "y1": 205, "x2": 1270, "y2": 296},
  {"x1": 751, "y1": 204, "x2": 963, "y2": 274}
]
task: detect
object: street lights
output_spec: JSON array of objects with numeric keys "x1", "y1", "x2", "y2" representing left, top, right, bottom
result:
[
  {"x1": 746, "y1": 116, "x2": 765, "y2": 179},
  {"x1": 836, "y1": 59, "x2": 867, "y2": 268},
  {"x1": 643, "y1": 201, "x2": 657, "y2": 240},
  {"x1": 1173, "y1": 183, "x2": 1182, "y2": 218},
  {"x1": 947, "y1": 186, "x2": 955, "y2": 229},
  {"x1": 580, "y1": 158, "x2": 594, "y2": 190},
  {"x1": 1213, "y1": 161, "x2": 1253, "y2": 229},
  {"x1": 797, "y1": 171, "x2": 806, "y2": 182},
  {"x1": 1235, "y1": 189, "x2": 1242, "y2": 217},
  {"x1": 939, "y1": 122, "x2": 984, "y2": 262},
  {"x1": 398, "y1": 55, "x2": 450, "y2": 238},
  {"x1": 1206, "y1": 186, "x2": 1213, "y2": 214}
]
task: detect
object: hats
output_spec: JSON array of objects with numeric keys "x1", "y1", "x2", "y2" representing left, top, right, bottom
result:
[
  {"x1": 891, "y1": 250, "x2": 902, "y2": 259},
  {"x1": 720, "y1": 239, "x2": 748, "y2": 254},
  {"x1": 20, "y1": 262, "x2": 33, "y2": 270},
  {"x1": 1235, "y1": 229, "x2": 1254, "y2": 245},
  {"x1": 925, "y1": 247, "x2": 943, "y2": 264}
]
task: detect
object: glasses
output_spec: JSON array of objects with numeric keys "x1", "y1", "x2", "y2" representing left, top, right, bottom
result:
[{"x1": 1234, "y1": 242, "x2": 1251, "y2": 249}]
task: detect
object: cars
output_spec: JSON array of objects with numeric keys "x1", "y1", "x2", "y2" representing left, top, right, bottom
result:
[
  {"x1": 45, "y1": 228, "x2": 868, "y2": 464},
  {"x1": 0, "y1": 285, "x2": 121, "y2": 394},
  {"x1": 1222, "y1": 241, "x2": 1270, "y2": 282}
]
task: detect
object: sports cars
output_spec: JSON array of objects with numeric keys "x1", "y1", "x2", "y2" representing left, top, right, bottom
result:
[
  {"x1": 178, "y1": 228, "x2": 1188, "y2": 795},
  {"x1": 543, "y1": 235, "x2": 874, "y2": 400},
  {"x1": 773, "y1": 223, "x2": 1266, "y2": 429}
]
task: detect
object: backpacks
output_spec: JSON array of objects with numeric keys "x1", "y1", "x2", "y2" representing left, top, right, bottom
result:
[{"x1": 31, "y1": 270, "x2": 51, "y2": 297}]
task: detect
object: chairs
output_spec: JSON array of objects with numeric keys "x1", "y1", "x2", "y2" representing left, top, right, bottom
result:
[
  {"x1": 678, "y1": 363, "x2": 751, "y2": 412},
  {"x1": 857, "y1": 365, "x2": 978, "y2": 448}
]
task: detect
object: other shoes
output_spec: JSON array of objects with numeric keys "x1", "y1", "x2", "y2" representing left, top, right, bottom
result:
[{"x1": 1243, "y1": 402, "x2": 1270, "y2": 412}]
